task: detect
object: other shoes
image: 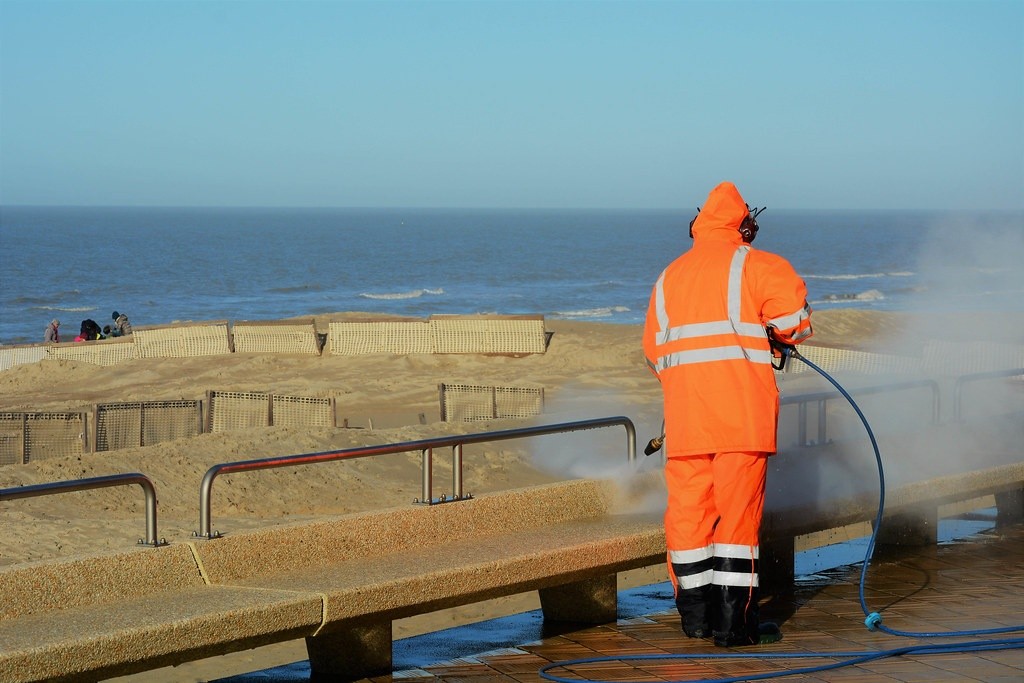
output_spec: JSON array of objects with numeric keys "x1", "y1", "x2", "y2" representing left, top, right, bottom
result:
[{"x1": 683, "y1": 621, "x2": 781, "y2": 648}]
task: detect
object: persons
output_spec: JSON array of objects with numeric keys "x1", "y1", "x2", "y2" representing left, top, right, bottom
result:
[
  {"x1": 44, "y1": 319, "x2": 61, "y2": 343},
  {"x1": 642, "y1": 180, "x2": 814, "y2": 647},
  {"x1": 74, "y1": 311, "x2": 131, "y2": 342}
]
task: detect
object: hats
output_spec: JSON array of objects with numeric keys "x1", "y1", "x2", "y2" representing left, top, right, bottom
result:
[
  {"x1": 112, "y1": 311, "x2": 119, "y2": 319},
  {"x1": 52, "y1": 319, "x2": 61, "y2": 325}
]
